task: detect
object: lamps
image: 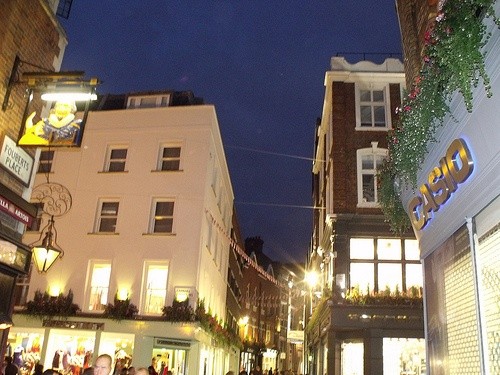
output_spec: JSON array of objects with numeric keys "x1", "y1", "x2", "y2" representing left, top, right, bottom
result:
[{"x1": 30, "y1": 215, "x2": 64, "y2": 273}]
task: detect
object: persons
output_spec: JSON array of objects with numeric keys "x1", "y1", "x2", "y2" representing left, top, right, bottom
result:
[
  {"x1": 13, "y1": 345, "x2": 27, "y2": 366},
  {"x1": 6, "y1": 342, "x2": 13, "y2": 362},
  {"x1": 3, "y1": 356, "x2": 13, "y2": 373},
  {"x1": 94, "y1": 353, "x2": 113, "y2": 374},
  {"x1": 120, "y1": 356, "x2": 176, "y2": 375},
  {"x1": 81, "y1": 351, "x2": 92, "y2": 375},
  {"x1": 32, "y1": 364, "x2": 44, "y2": 375},
  {"x1": 226, "y1": 365, "x2": 301, "y2": 375},
  {"x1": 113, "y1": 348, "x2": 134, "y2": 375},
  {"x1": 43, "y1": 369, "x2": 60, "y2": 375},
  {"x1": 53, "y1": 347, "x2": 68, "y2": 375},
  {"x1": 84, "y1": 367, "x2": 95, "y2": 375},
  {"x1": 5, "y1": 363, "x2": 20, "y2": 375}
]
